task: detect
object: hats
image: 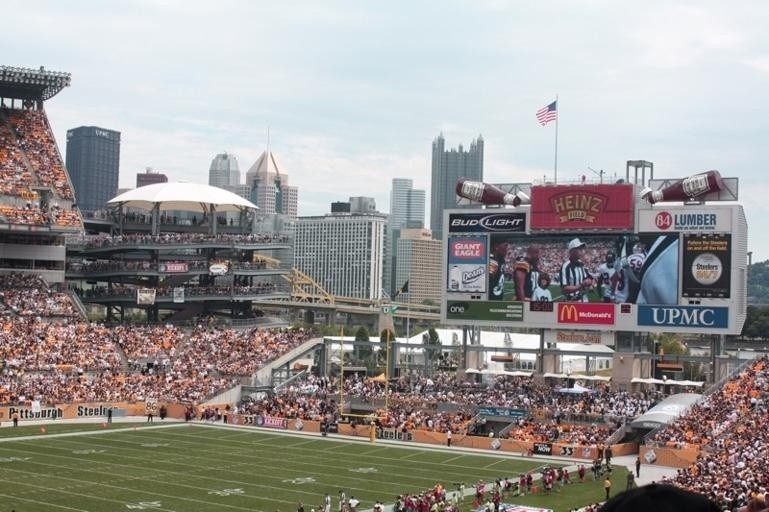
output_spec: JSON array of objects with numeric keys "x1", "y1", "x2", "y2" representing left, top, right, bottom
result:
[{"x1": 568, "y1": 237, "x2": 586, "y2": 253}]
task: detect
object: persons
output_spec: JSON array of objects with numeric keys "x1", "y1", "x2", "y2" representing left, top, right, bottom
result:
[
  {"x1": 0, "y1": 105, "x2": 83, "y2": 209},
  {"x1": 11, "y1": 411, "x2": 19, "y2": 428},
  {"x1": 294, "y1": 326, "x2": 769, "y2": 511},
  {"x1": 488, "y1": 233, "x2": 680, "y2": 307},
  {"x1": 0, "y1": 210, "x2": 294, "y2": 425}
]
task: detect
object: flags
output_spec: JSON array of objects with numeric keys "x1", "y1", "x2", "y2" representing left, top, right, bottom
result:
[{"x1": 534, "y1": 99, "x2": 557, "y2": 128}]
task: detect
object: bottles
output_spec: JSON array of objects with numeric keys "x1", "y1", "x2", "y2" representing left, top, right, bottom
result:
[
  {"x1": 648, "y1": 170, "x2": 725, "y2": 204},
  {"x1": 454, "y1": 177, "x2": 521, "y2": 207},
  {"x1": 448, "y1": 265, "x2": 462, "y2": 291}
]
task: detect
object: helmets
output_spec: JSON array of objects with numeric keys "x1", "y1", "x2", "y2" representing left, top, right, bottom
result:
[
  {"x1": 538, "y1": 273, "x2": 552, "y2": 290},
  {"x1": 606, "y1": 251, "x2": 616, "y2": 270}
]
